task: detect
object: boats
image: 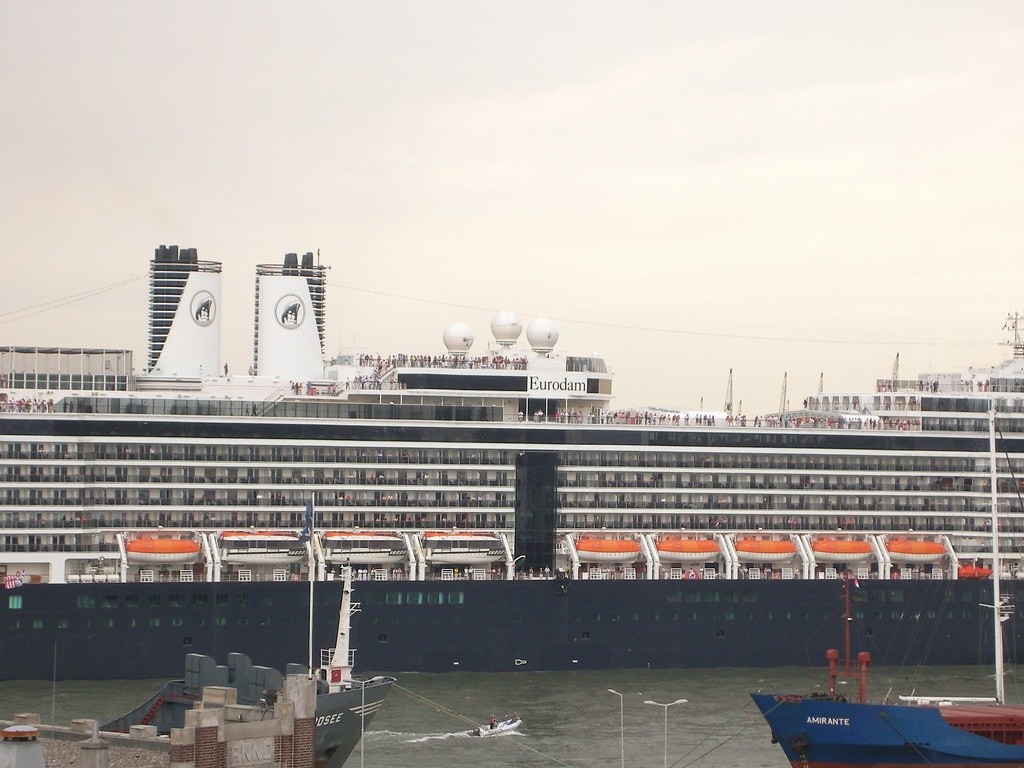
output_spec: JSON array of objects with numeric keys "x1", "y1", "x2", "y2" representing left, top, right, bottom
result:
[
  {"x1": 477, "y1": 718, "x2": 522, "y2": 738},
  {"x1": 98, "y1": 558, "x2": 399, "y2": 768},
  {"x1": 748, "y1": 392, "x2": 1024, "y2": 768}
]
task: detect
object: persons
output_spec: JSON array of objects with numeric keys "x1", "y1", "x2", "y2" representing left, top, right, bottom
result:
[
  {"x1": 1, "y1": 397, "x2": 723, "y2": 584},
  {"x1": 725, "y1": 379, "x2": 1024, "y2": 581},
  {"x1": 489, "y1": 713, "x2": 498, "y2": 728},
  {"x1": 290, "y1": 354, "x2": 527, "y2": 395}
]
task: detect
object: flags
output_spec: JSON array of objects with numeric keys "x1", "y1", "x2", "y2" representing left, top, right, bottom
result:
[{"x1": 296, "y1": 499, "x2": 313, "y2": 543}]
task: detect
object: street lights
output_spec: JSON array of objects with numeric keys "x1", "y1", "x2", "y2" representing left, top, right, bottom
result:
[
  {"x1": 607, "y1": 688, "x2": 625, "y2": 768},
  {"x1": 340, "y1": 676, "x2": 387, "y2": 768},
  {"x1": 643, "y1": 698, "x2": 688, "y2": 768}
]
